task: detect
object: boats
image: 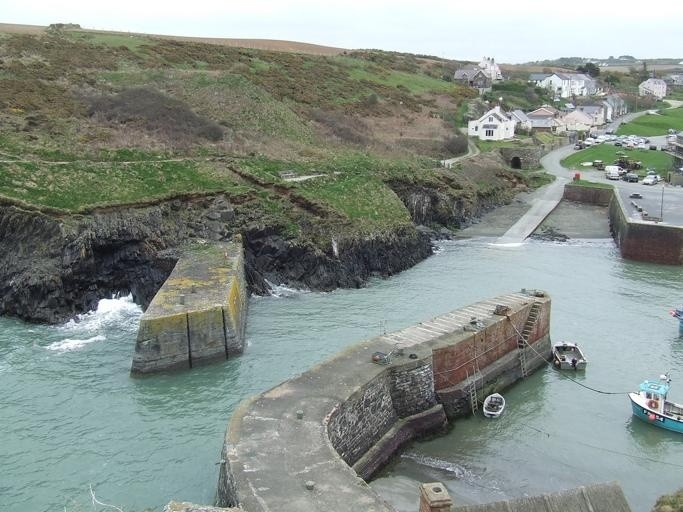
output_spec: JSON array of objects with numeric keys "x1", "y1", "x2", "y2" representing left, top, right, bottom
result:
[
  {"x1": 670, "y1": 308, "x2": 682, "y2": 321},
  {"x1": 625, "y1": 369, "x2": 682, "y2": 437},
  {"x1": 552, "y1": 340, "x2": 587, "y2": 371}
]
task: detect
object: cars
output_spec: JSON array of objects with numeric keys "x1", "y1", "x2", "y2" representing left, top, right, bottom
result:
[{"x1": 573, "y1": 128, "x2": 661, "y2": 199}]
